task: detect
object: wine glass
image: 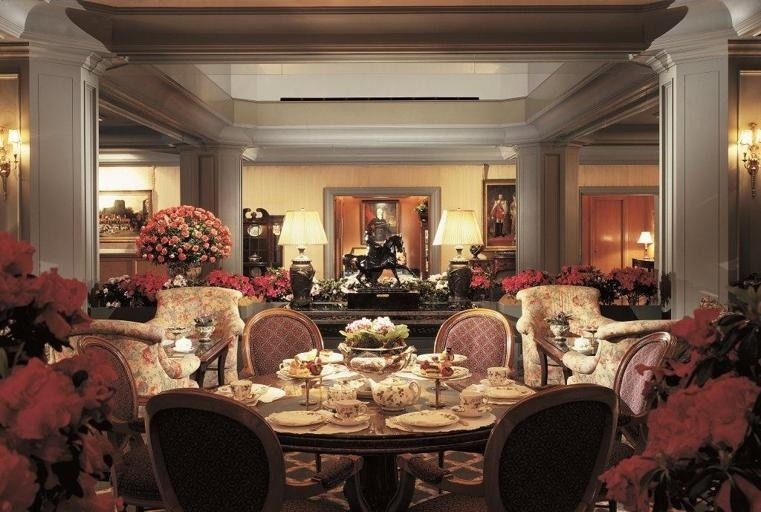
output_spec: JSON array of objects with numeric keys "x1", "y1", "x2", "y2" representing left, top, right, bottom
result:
[{"x1": 583, "y1": 329, "x2": 600, "y2": 345}]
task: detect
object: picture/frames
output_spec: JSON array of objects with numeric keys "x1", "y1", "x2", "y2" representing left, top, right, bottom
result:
[
  {"x1": 98, "y1": 189, "x2": 153, "y2": 243},
  {"x1": 359, "y1": 199, "x2": 400, "y2": 245},
  {"x1": 482, "y1": 179, "x2": 516, "y2": 252}
]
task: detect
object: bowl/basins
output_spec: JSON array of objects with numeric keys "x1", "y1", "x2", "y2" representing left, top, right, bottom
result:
[{"x1": 247, "y1": 256, "x2": 262, "y2": 263}]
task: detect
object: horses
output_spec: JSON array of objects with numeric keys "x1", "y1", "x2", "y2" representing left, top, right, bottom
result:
[{"x1": 341, "y1": 234, "x2": 417, "y2": 289}]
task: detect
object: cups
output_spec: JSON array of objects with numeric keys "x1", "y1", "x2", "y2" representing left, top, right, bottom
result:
[
  {"x1": 230, "y1": 380, "x2": 253, "y2": 402},
  {"x1": 460, "y1": 392, "x2": 489, "y2": 411},
  {"x1": 488, "y1": 366, "x2": 508, "y2": 385},
  {"x1": 176, "y1": 336, "x2": 193, "y2": 350},
  {"x1": 574, "y1": 335, "x2": 590, "y2": 348},
  {"x1": 336, "y1": 399, "x2": 368, "y2": 419}
]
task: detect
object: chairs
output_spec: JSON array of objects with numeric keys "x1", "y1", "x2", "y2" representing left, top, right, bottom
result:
[
  {"x1": 560, "y1": 317, "x2": 679, "y2": 387},
  {"x1": 145, "y1": 388, "x2": 368, "y2": 510},
  {"x1": 145, "y1": 289, "x2": 247, "y2": 389},
  {"x1": 74, "y1": 337, "x2": 202, "y2": 512},
  {"x1": 610, "y1": 330, "x2": 675, "y2": 465},
  {"x1": 392, "y1": 381, "x2": 619, "y2": 512},
  {"x1": 431, "y1": 307, "x2": 514, "y2": 494},
  {"x1": 48, "y1": 319, "x2": 201, "y2": 436},
  {"x1": 241, "y1": 308, "x2": 326, "y2": 479},
  {"x1": 517, "y1": 285, "x2": 618, "y2": 387}
]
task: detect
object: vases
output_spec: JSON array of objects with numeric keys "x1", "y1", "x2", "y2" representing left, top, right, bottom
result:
[
  {"x1": 549, "y1": 322, "x2": 571, "y2": 340},
  {"x1": 338, "y1": 342, "x2": 416, "y2": 376}
]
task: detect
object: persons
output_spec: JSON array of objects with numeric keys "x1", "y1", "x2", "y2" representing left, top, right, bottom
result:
[
  {"x1": 510, "y1": 193, "x2": 518, "y2": 235},
  {"x1": 366, "y1": 208, "x2": 393, "y2": 241},
  {"x1": 364, "y1": 227, "x2": 383, "y2": 275},
  {"x1": 490, "y1": 193, "x2": 509, "y2": 237}
]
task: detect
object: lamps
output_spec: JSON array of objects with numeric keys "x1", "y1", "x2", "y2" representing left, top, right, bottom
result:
[
  {"x1": 0, "y1": 130, "x2": 21, "y2": 199},
  {"x1": 431, "y1": 209, "x2": 487, "y2": 305},
  {"x1": 275, "y1": 209, "x2": 329, "y2": 310},
  {"x1": 738, "y1": 127, "x2": 761, "y2": 199},
  {"x1": 637, "y1": 232, "x2": 655, "y2": 261}
]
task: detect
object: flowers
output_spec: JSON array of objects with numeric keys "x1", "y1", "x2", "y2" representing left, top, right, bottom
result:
[
  {"x1": 338, "y1": 311, "x2": 410, "y2": 349},
  {"x1": 459, "y1": 265, "x2": 657, "y2": 306},
  {"x1": 84, "y1": 202, "x2": 294, "y2": 310},
  {"x1": 595, "y1": 283, "x2": 761, "y2": 512},
  {"x1": 544, "y1": 311, "x2": 573, "y2": 326},
  {"x1": 1, "y1": 238, "x2": 125, "y2": 512}
]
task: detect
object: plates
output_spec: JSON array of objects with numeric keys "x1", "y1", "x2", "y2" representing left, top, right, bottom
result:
[
  {"x1": 247, "y1": 224, "x2": 263, "y2": 237},
  {"x1": 572, "y1": 347, "x2": 594, "y2": 351},
  {"x1": 451, "y1": 405, "x2": 494, "y2": 417},
  {"x1": 274, "y1": 343, "x2": 470, "y2": 404},
  {"x1": 218, "y1": 383, "x2": 269, "y2": 394},
  {"x1": 173, "y1": 347, "x2": 195, "y2": 353},
  {"x1": 266, "y1": 410, "x2": 326, "y2": 426},
  {"x1": 250, "y1": 267, "x2": 264, "y2": 277},
  {"x1": 328, "y1": 413, "x2": 371, "y2": 426},
  {"x1": 486, "y1": 386, "x2": 532, "y2": 399},
  {"x1": 399, "y1": 411, "x2": 460, "y2": 427},
  {"x1": 480, "y1": 379, "x2": 515, "y2": 386}
]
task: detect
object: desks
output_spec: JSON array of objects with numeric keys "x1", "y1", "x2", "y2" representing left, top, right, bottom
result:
[
  {"x1": 528, "y1": 335, "x2": 602, "y2": 389},
  {"x1": 154, "y1": 330, "x2": 232, "y2": 391},
  {"x1": 629, "y1": 258, "x2": 654, "y2": 275}
]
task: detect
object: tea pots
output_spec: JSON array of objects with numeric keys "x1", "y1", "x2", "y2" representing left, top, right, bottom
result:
[{"x1": 367, "y1": 376, "x2": 421, "y2": 411}]
